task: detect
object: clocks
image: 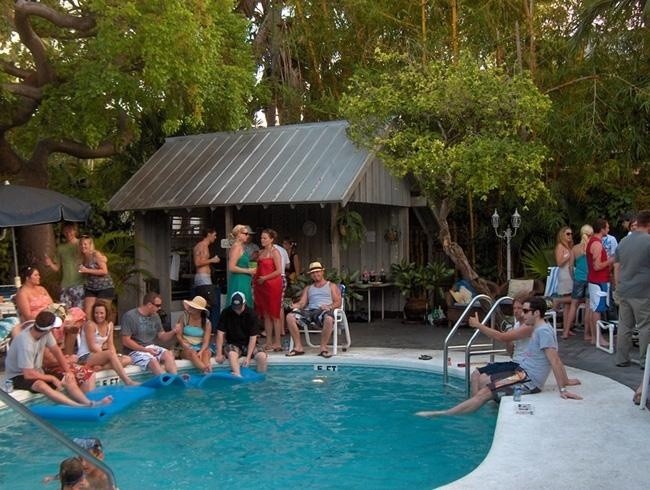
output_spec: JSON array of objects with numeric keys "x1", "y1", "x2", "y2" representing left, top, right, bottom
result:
[{"x1": 302, "y1": 221, "x2": 317, "y2": 237}]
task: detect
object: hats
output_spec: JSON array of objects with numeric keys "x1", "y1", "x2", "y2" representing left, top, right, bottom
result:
[
  {"x1": 306, "y1": 261, "x2": 326, "y2": 273},
  {"x1": 182, "y1": 296, "x2": 210, "y2": 316},
  {"x1": 229, "y1": 292, "x2": 246, "y2": 310},
  {"x1": 35, "y1": 316, "x2": 63, "y2": 331}
]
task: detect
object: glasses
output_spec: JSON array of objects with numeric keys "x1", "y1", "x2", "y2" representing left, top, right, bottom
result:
[
  {"x1": 240, "y1": 232, "x2": 249, "y2": 236},
  {"x1": 151, "y1": 302, "x2": 163, "y2": 308},
  {"x1": 566, "y1": 233, "x2": 574, "y2": 235},
  {"x1": 523, "y1": 308, "x2": 540, "y2": 313}
]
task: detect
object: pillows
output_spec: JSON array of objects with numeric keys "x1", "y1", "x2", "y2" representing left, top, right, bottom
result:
[
  {"x1": 449, "y1": 286, "x2": 473, "y2": 304},
  {"x1": 507, "y1": 279, "x2": 534, "y2": 301}
]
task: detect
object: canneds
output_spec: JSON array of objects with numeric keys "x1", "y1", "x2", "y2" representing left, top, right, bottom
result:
[{"x1": 514, "y1": 385, "x2": 522, "y2": 401}]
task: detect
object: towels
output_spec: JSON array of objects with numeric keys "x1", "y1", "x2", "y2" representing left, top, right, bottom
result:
[{"x1": 171, "y1": 252, "x2": 181, "y2": 281}]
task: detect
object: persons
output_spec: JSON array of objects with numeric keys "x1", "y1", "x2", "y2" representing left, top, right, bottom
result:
[
  {"x1": 414, "y1": 296, "x2": 583, "y2": 416},
  {"x1": 43, "y1": 438, "x2": 110, "y2": 490},
  {"x1": 468, "y1": 289, "x2": 558, "y2": 398},
  {"x1": 555, "y1": 211, "x2": 650, "y2": 369},
  {"x1": 59, "y1": 457, "x2": 89, "y2": 490}
]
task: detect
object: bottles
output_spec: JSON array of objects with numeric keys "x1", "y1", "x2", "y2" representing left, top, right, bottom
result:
[
  {"x1": 362, "y1": 263, "x2": 385, "y2": 284},
  {"x1": 514, "y1": 381, "x2": 522, "y2": 401}
]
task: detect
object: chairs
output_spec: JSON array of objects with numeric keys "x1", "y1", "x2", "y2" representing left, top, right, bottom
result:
[
  {"x1": 288, "y1": 284, "x2": 351, "y2": 355},
  {"x1": 541, "y1": 267, "x2": 650, "y2": 410},
  {"x1": 495, "y1": 277, "x2": 545, "y2": 316},
  {"x1": 0, "y1": 285, "x2": 21, "y2": 319},
  {"x1": 446, "y1": 280, "x2": 500, "y2": 328}
]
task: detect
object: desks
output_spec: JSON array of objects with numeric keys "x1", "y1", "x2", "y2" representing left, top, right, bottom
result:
[{"x1": 349, "y1": 282, "x2": 405, "y2": 324}]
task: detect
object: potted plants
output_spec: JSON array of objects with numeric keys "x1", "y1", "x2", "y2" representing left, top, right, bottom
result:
[
  {"x1": 384, "y1": 223, "x2": 401, "y2": 242},
  {"x1": 336, "y1": 207, "x2": 367, "y2": 251},
  {"x1": 390, "y1": 256, "x2": 456, "y2": 325}
]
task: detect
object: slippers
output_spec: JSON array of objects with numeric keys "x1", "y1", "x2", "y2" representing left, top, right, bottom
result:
[
  {"x1": 318, "y1": 351, "x2": 331, "y2": 358},
  {"x1": 285, "y1": 349, "x2": 306, "y2": 357}
]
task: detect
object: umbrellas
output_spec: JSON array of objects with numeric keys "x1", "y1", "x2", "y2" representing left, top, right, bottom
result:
[{"x1": 0, "y1": 180, "x2": 93, "y2": 278}]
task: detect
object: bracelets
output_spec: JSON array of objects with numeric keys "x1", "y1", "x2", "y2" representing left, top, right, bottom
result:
[{"x1": 559, "y1": 386, "x2": 566, "y2": 393}]
